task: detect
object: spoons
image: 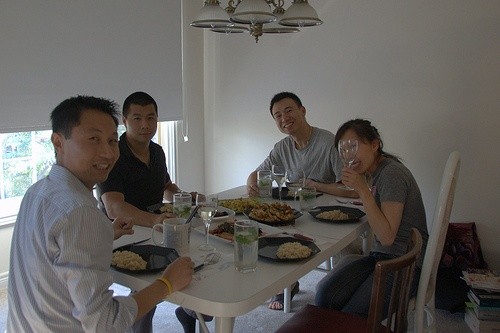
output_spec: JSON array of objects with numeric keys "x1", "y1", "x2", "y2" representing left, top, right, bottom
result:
[{"x1": 192, "y1": 253, "x2": 215, "y2": 272}]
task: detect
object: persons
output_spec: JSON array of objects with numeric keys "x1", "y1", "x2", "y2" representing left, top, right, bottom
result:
[
  {"x1": 7, "y1": 96, "x2": 193, "y2": 333},
  {"x1": 313, "y1": 119, "x2": 429, "y2": 323},
  {"x1": 96, "y1": 91, "x2": 213, "y2": 333},
  {"x1": 247, "y1": 93, "x2": 362, "y2": 310}
]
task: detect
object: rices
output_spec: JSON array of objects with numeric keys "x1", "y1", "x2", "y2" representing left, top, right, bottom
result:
[
  {"x1": 160, "y1": 203, "x2": 174, "y2": 213},
  {"x1": 276, "y1": 241, "x2": 313, "y2": 259},
  {"x1": 111, "y1": 250, "x2": 148, "y2": 270},
  {"x1": 316, "y1": 210, "x2": 348, "y2": 219}
]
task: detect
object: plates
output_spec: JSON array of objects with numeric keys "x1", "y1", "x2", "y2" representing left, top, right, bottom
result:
[
  {"x1": 274, "y1": 186, "x2": 322, "y2": 199},
  {"x1": 213, "y1": 206, "x2": 235, "y2": 222},
  {"x1": 110, "y1": 244, "x2": 181, "y2": 273},
  {"x1": 308, "y1": 205, "x2": 366, "y2": 223},
  {"x1": 243, "y1": 207, "x2": 303, "y2": 226},
  {"x1": 235, "y1": 198, "x2": 257, "y2": 216},
  {"x1": 258, "y1": 236, "x2": 321, "y2": 261},
  {"x1": 194, "y1": 218, "x2": 283, "y2": 244}
]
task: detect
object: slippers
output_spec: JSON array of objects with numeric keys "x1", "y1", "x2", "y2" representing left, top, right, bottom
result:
[{"x1": 271, "y1": 281, "x2": 300, "y2": 310}]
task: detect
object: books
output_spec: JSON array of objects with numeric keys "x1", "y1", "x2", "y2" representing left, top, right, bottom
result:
[{"x1": 460, "y1": 268, "x2": 500, "y2": 333}]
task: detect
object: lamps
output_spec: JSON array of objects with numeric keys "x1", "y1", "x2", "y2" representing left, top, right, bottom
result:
[{"x1": 189, "y1": 0, "x2": 324, "y2": 42}]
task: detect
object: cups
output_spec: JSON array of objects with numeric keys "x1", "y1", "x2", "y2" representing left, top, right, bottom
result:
[
  {"x1": 257, "y1": 170, "x2": 272, "y2": 200},
  {"x1": 172, "y1": 193, "x2": 192, "y2": 218},
  {"x1": 298, "y1": 186, "x2": 316, "y2": 211},
  {"x1": 151, "y1": 218, "x2": 190, "y2": 256},
  {"x1": 234, "y1": 220, "x2": 259, "y2": 273}
]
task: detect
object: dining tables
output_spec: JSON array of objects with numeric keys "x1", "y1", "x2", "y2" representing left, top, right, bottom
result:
[{"x1": 109, "y1": 180, "x2": 374, "y2": 333}]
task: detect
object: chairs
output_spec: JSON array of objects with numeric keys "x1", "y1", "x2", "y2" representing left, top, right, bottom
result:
[{"x1": 273, "y1": 149, "x2": 462, "y2": 333}]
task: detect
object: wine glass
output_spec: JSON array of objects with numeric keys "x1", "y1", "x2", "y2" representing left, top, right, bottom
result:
[
  {"x1": 284, "y1": 167, "x2": 305, "y2": 212},
  {"x1": 338, "y1": 139, "x2": 359, "y2": 191},
  {"x1": 195, "y1": 193, "x2": 218, "y2": 251},
  {"x1": 272, "y1": 160, "x2": 288, "y2": 204}
]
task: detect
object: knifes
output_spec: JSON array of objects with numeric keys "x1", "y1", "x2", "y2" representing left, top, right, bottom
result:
[{"x1": 113, "y1": 237, "x2": 151, "y2": 250}]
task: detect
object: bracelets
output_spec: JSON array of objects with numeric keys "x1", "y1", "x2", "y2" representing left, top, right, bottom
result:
[{"x1": 156, "y1": 278, "x2": 173, "y2": 293}]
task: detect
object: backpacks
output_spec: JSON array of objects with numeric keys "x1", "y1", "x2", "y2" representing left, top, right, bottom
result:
[{"x1": 436, "y1": 223, "x2": 487, "y2": 312}]
